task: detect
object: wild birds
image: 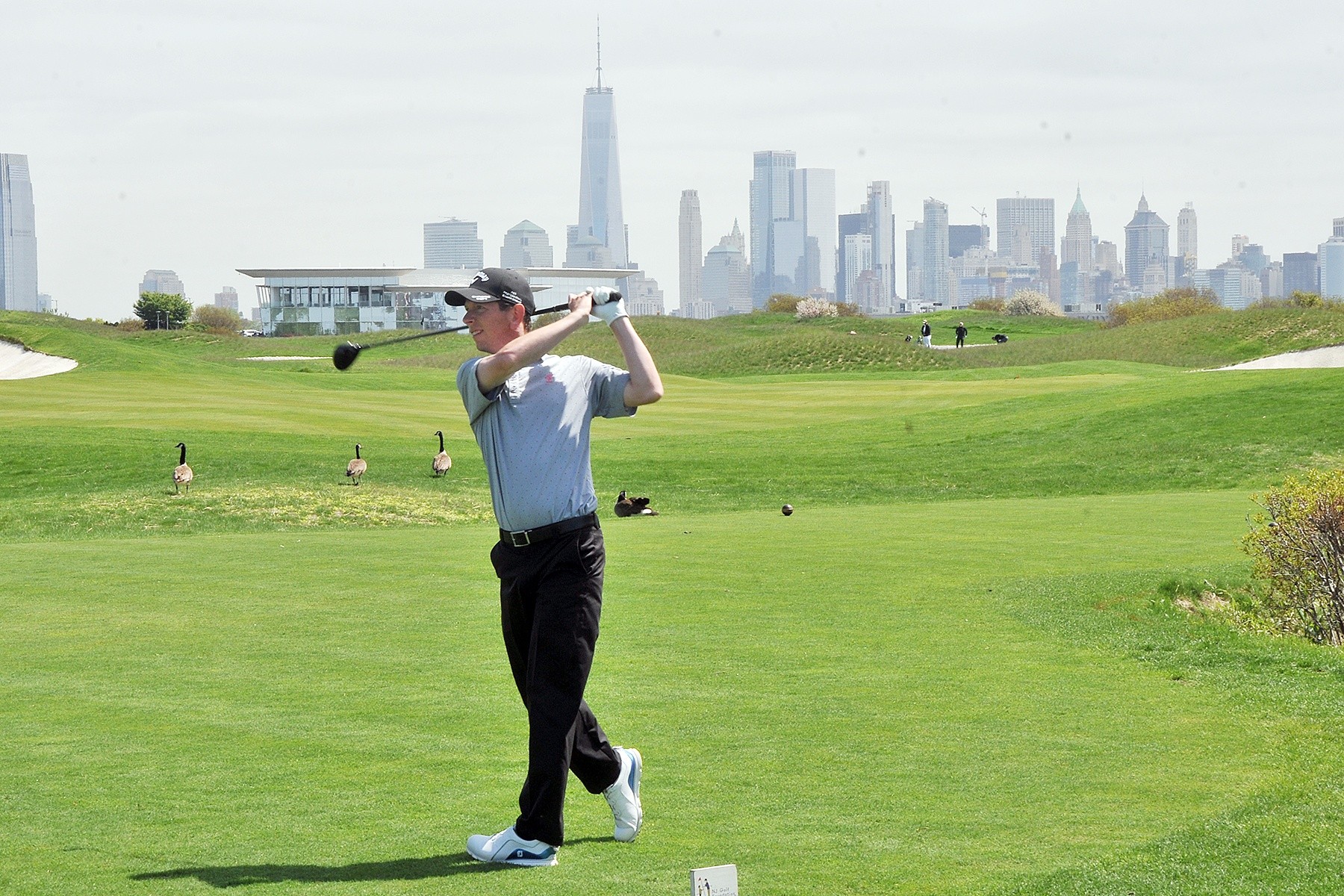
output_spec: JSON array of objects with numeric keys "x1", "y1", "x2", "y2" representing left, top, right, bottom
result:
[
  {"x1": 431, "y1": 430, "x2": 452, "y2": 478},
  {"x1": 613, "y1": 490, "x2": 660, "y2": 518},
  {"x1": 346, "y1": 443, "x2": 367, "y2": 486},
  {"x1": 172, "y1": 442, "x2": 193, "y2": 494}
]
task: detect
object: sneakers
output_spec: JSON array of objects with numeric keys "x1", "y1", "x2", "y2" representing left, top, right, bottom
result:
[
  {"x1": 465, "y1": 822, "x2": 560, "y2": 866},
  {"x1": 602, "y1": 746, "x2": 642, "y2": 842}
]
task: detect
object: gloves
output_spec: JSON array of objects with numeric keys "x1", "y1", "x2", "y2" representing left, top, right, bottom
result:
[{"x1": 585, "y1": 286, "x2": 629, "y2": 327}]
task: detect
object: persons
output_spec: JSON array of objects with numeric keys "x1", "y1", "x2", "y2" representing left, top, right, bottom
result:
[
  {"x1": 443, "y1": 266, "x2": 666, "y2": 868},
  {"x1": 991, "y1": 334, "x2": 1009, "y2": 345},
  {"x1": 904, "y1": 319, "x2": 932, "y2": 349},
  {"x1": 955, "y1": 321, "x2": 968, "y2": 349}
]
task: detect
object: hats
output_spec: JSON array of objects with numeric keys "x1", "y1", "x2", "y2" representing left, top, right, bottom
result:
[
  {"x1": 445, "y1": 268, "x2": 535, "y2": 316},
  {"x1": 923, "y1": 320, "x2": 927, "y2": 323}
]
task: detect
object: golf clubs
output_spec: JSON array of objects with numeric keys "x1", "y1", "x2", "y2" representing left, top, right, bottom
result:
[{"x1": 333, "y1": 291, "x2": 622, "y2": 371}]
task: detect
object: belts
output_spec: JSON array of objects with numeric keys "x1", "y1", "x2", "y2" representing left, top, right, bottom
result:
[{"x1": 499, "y1": 513, "x2": 599, "y2": 547}]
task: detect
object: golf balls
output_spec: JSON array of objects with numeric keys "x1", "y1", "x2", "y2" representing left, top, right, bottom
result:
[{"x1": 782, "y1": 504, "x2": 794, "y2": 515}]
